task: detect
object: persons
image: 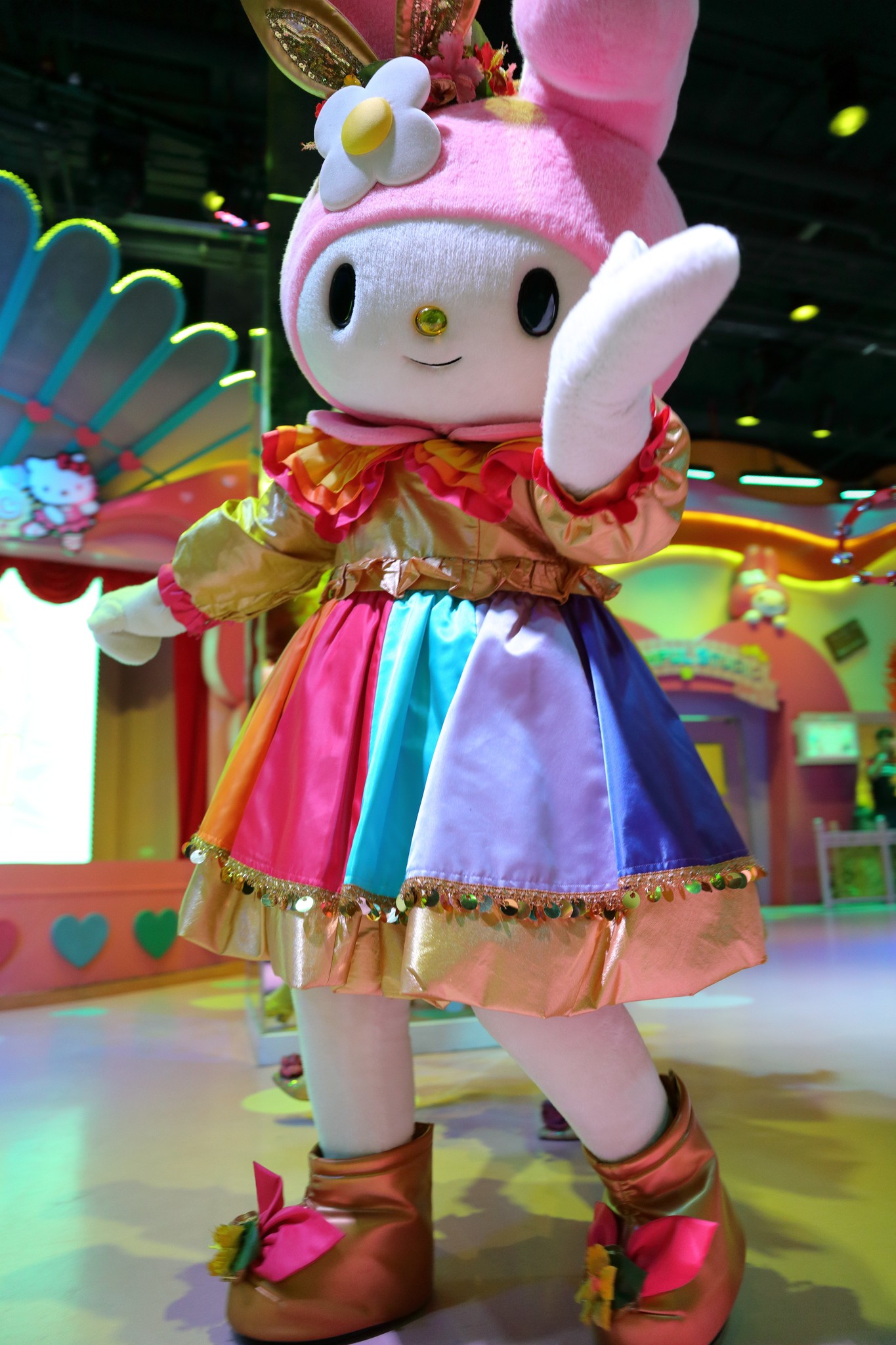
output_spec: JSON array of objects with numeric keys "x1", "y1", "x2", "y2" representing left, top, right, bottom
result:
[{"x1": 864, "y1": 728, "x2": 896, "y2": 828}]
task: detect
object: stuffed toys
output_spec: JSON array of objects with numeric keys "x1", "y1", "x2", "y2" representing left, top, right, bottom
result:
[{"x1": 86, "y1": 0, "x2": 772, "y2": 1345}]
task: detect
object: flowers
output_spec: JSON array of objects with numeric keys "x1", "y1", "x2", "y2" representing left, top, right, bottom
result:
[
  {"x1": 204, "y1": 1209, "x2": 261, "y2": 1277},
  {"x1": 575, "y1": 1243, "x2": 648, "y2": 1332},
  {"x1": 315, "y1": 31, "x2": 518, "y2": 121}
]
